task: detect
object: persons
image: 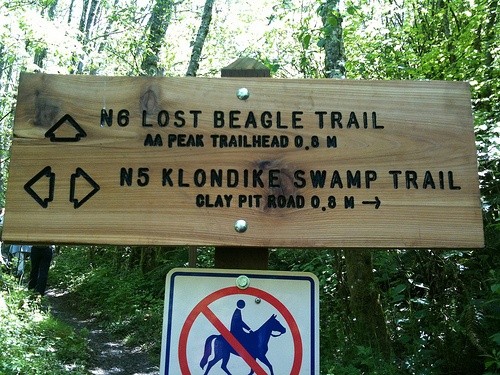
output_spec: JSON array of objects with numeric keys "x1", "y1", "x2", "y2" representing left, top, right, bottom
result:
[{"x1": 0, "y1": 205, "x2": 54, "y2": 298}]
